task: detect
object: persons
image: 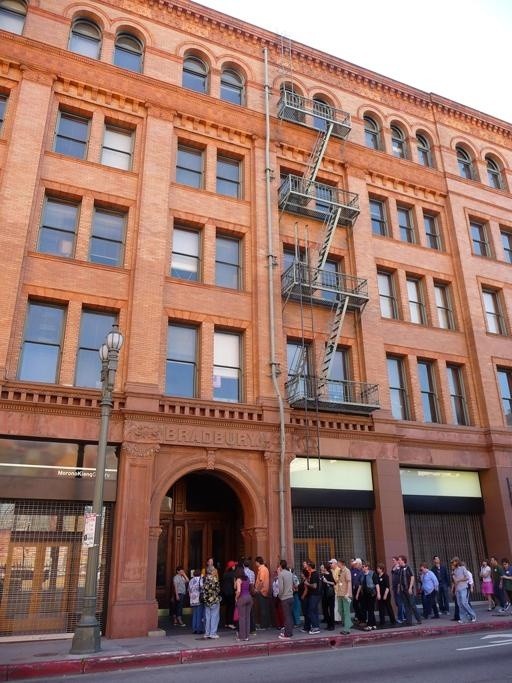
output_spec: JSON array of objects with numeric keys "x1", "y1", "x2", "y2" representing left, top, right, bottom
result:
[{"x1": 170, "y1": 553, "x2": 511, "y2": 642}]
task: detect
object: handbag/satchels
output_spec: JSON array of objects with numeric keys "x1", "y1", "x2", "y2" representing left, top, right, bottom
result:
[{"x1": 232, "y1": 603, "x2": 240, "y2": 622}]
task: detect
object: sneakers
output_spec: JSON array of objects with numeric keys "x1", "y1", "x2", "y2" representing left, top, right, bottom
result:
[
  {"x1": 299, "y1": 628, "x2": 320, "y2": 635},
  {"x1": 278, "y1": 627, "x2": 291, "y2": 639}
]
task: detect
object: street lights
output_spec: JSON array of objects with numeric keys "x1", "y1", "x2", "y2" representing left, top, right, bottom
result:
[{"x1": 70, "y1": 323, "x2": 130, "y2": 655}]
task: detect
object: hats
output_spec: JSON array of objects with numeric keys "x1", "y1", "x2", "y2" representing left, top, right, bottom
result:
[
  {"x1": 450, "y1": 557, "x2": 459, "y2": 562},
  {"x1": 226, "y1": 560, "x2": 238, "y2": 568},
  {"x1": 328, "y1": 558, "x2": 338, "y2": 563}
]
